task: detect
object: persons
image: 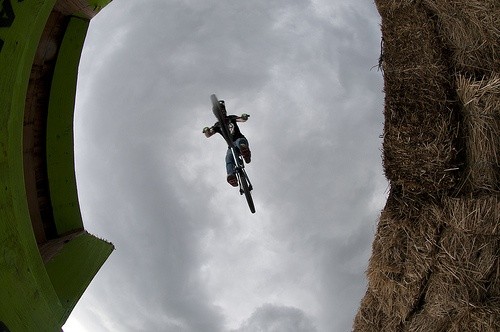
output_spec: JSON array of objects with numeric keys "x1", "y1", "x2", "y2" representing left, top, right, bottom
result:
[{"x1": 203, "y1": 103, "x2": 252, "y2": 186}]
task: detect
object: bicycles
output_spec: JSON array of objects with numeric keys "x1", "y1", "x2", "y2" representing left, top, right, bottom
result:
[{"x1": 201, "y1": 93, "x2": 257, "y2": 214}]
